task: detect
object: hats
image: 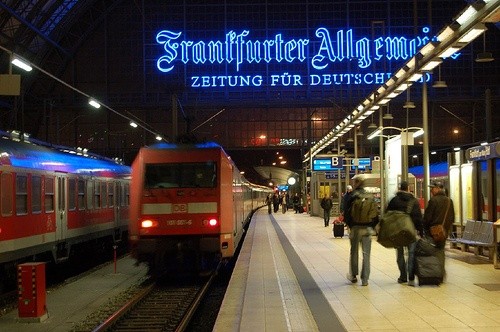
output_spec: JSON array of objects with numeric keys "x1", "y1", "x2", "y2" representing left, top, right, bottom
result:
[
  {"x1": 428, "y1": 183, "x2": 443, "y2": 188},
  {"x1": 351, "y1": 174, "x2": 365, "y2": 181}
]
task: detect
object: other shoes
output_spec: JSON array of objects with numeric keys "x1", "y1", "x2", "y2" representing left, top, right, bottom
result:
[
  {"x1": 408, "y1": 280, "x2": 416, "y2": 286},
  {"x1": 398, "y1": 277, "x2": 407, "y2": 283},
  {"x1": 346, "y1": 273, "x2": 357, "y2": 283},
  {"x1": 362, "y1": 280, "x2": 368, "y2": 286}
]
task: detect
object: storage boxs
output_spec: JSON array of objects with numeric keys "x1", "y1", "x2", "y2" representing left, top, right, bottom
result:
[{"x1": 334, "y1": 223, "x2": 345, "y2": 239}]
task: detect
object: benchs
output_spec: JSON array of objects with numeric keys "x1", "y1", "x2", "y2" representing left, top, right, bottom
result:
[{"x1": 446, "y1": 219, "x2": 496, "y2": 261}]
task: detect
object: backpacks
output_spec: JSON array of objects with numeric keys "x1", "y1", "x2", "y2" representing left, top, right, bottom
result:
[{"x1": 351, "y1": 191, "x2": 376, "y2": 223}]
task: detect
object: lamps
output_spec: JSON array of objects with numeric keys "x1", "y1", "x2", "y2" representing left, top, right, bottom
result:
[
  {"x1": 9, "y1": 54, "x2": 33, "y2": 72},
  {"x1": 130, "y1": 120, "x2": 138, "y2": 128},
  {"x1": 88, "y1": 96, "x2": 102, "y2": 109},
  {"x1": 155, "y1": 134, "x2": 163, "y2": 141},
  {"x1": 303, "y1": 0, "x2": 500, "y2": 163}
]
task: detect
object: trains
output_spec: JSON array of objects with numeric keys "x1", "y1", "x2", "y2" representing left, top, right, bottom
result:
[
  {"x1": 126, "y1": 145, "x2": 274, "y2": 264},
  {"x1": 0, "y1": 141, "x2": 134, "y2": 270}
]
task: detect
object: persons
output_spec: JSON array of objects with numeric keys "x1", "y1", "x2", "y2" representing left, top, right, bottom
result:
[
  {"x1": 383, "y1": 181, "x2": 422, "y2": 286},
  {"x1": 424, "y1": 181, "x2": 455, "y2": 280},
  {"x1": 342, "y1": 177, "x2": 371, "y2": 286},
  {"x1": 273, "y1": 193, "x2": 289, "y2": 213},
  {"x1": 340, "y1": 185, "x2": 352, "y2": 215},
  {"x1": 321, "y1": 194, "x2": 333, "y2": 227},
  {"x1": 267, "y1": 195, "x2": 269, "y2": 200},
  {"x1": 293, "y1": 193, "x2": 299, "y2": 214}
]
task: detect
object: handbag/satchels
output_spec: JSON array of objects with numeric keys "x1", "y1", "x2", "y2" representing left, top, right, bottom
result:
[
  {"x1": 377, "y1": 199, "x2": 417, "y2": 248},
  {"x1": 430, "y1": 224, "x2": 446, "y2": 242}
]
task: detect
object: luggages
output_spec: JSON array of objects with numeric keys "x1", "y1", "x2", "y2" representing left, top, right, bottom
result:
[
  {"x1": 333, "y1": 213, "x2": 344, "y2": 238},
  {"x1": 415, "y1": 238, "x2": 445, "y2": 286}
]
